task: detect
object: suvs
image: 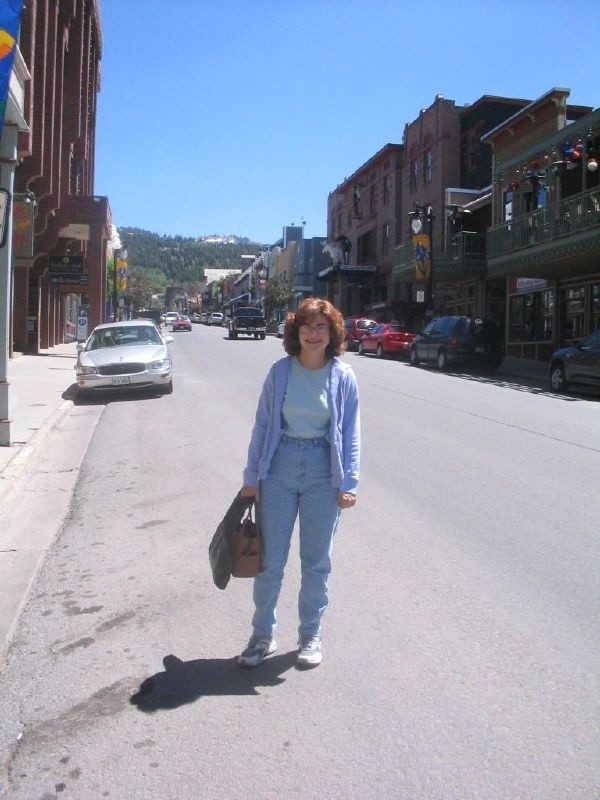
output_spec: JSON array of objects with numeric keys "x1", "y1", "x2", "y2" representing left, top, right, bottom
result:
[{"x1": 410, "y1": 315, "x2": 507, "y2": 375}]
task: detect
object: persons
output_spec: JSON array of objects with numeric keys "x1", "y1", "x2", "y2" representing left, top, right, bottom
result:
[{"x1": 235, "y1": 295, "x2": 360, "y2": 668}]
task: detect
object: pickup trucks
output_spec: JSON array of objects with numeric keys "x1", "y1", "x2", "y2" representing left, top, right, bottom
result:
[{"x1": 227, "y1": 306, "x2": 266, "y2": 340}]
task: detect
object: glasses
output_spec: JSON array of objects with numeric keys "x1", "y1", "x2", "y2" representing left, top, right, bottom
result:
[{"x1": 300, "y1": 324, "x2": 330, "y2": 335}]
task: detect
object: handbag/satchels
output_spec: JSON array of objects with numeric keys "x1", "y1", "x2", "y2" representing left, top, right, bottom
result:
[
  {"x1": 231, "y1": 504, "x2": 261, "y2": 578},
  {"x1": 208, "y1": 488, "x2": 255, "y2": 590}
]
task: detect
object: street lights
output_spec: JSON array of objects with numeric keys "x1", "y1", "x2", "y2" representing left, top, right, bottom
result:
[{"x1": 407, "y1": 201, "x2": 433, "y2": 323}]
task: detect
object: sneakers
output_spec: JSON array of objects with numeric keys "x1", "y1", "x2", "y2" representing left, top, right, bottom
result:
[
  {"x1": 238, "y1": 635, "x2": 278, "y2": 666},
  {"x1": 296, "y1": 626, "x2": 323, "y2": 665}
]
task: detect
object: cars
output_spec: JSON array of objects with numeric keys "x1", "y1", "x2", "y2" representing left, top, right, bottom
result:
[
  {"x1": 159, "y1": 312, "x2": 192, "y2": 331},
  {"x1": 75, "y1": 310, "x2": 173, "y2": 400},
  {"x1": 342, "y1": 319, "x2": 417, "y2": 359},
  {"x1": 547, "y1": 330, "x2": 600, "y2": 392},
  {"x1": 189, "y1": 312, "x2": 223, "y2": 326}
]
